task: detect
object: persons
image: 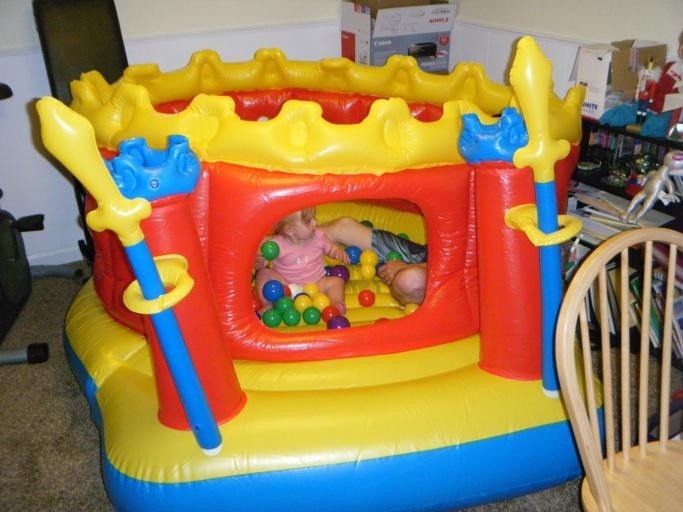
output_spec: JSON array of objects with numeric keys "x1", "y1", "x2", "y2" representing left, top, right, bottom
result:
[{"x1": 254, "y1": 202, "x2": 427, "y2": 327}]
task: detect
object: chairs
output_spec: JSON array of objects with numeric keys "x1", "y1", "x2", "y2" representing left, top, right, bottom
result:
[{"x1": 549, "y1": 224, "x2": 683, "y2": 512}]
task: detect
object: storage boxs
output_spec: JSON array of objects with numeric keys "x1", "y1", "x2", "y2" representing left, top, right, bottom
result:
[
  {"x1": 341, "y1": 1, "x2": 452, "y2": 81},
  {"x1": 570, "y1": 36, "x2": 669, "y2": 112}
]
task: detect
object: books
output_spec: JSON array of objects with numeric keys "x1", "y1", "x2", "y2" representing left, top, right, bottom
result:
[{"x1": 552, "y1": 132, "x2": 682, "y2": 374}]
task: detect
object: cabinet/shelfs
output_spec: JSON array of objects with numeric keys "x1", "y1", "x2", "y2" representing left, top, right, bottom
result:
[{"x1": 566, "y1": 110, "x2": 682, "y2": 368}]
